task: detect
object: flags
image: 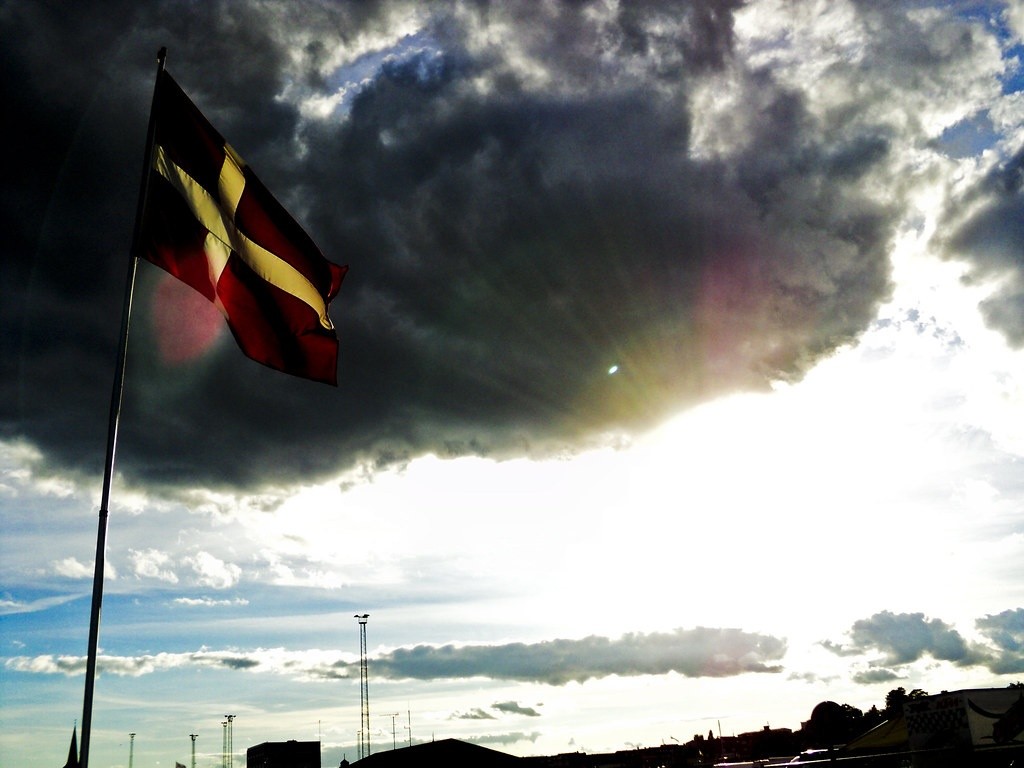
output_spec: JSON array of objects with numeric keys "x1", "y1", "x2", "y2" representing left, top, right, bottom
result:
[{"x1": 129, "y1": 68, "x2": 351, "y2": 388}]
